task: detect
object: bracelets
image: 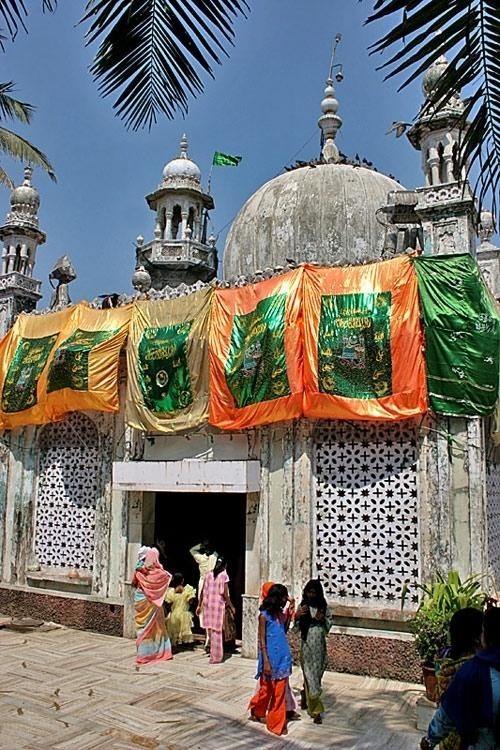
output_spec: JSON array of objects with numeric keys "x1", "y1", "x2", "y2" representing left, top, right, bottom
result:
[{"x1": 260, "y1": 647, "x2": 267, "y2": 650}]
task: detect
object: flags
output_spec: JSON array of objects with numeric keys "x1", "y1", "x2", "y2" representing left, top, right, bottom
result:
[{"x1": 211, "y1": 150, "x2": 245, "y2": 169}]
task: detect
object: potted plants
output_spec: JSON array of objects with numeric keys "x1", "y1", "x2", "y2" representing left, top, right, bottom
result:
[{"x1": 408, "y1": 607, "x2": 452, "y2": 700}]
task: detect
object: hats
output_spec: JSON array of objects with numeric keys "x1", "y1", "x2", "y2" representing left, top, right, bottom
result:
[{"x1": 138, "y1": 546, "x2": 151, "y2": 560}]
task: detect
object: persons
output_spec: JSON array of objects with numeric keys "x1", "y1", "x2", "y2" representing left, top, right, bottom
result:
[
  {"x1": 248, "y1": 584, "x2": 296, "y2": 736},
  {"x1": 133, "y1": 546, "x2": 153, "y2": 576},
  {"x1": 291, "y1": 580, "x2": 334, "y2": 724},
  {"x1": 131, "y1": 546, "x2": 173, "y2": 665},
  {"x1": 438, "y1": 607, "x2": 487, "y2": 750},
  {"x1": 164, "y1": 572, "x2": 197, "y2": 651},
  {"x1": 189, "y1": 537, "x2": 220, "y2": 613},
  {"x1": 420, "y1": 608, "x2": 499, "y2": 750},
  {"x1": 196, "y1": 555, "x2": 237, "y2": 665}
]
